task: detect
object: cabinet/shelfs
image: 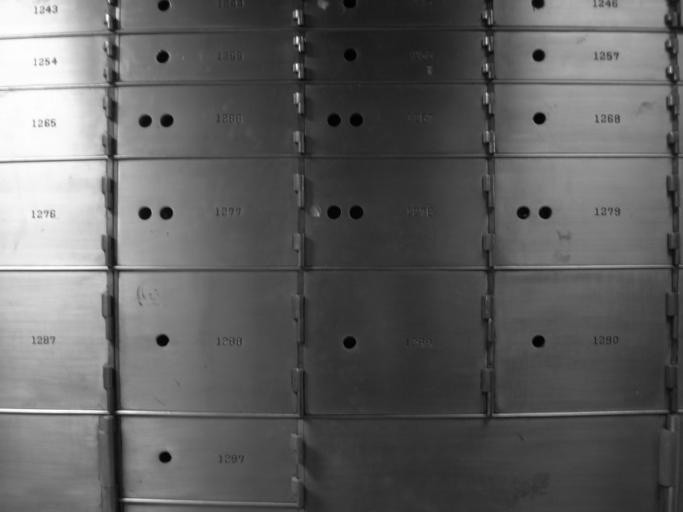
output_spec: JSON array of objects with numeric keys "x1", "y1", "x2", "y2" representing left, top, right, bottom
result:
[{"x1": 0, "y1": 0, "x2": 683, "y2": 511}]
